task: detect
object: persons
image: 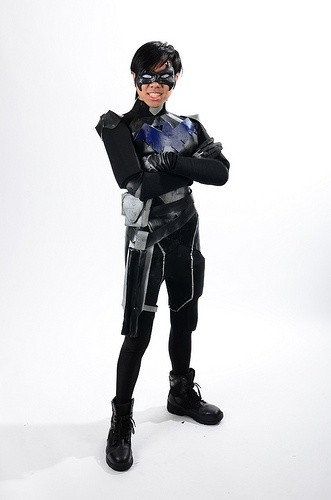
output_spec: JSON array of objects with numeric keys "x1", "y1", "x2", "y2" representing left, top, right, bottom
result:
[{"x1": 96, "y1": 41, "x2": 230, "y2": 474}]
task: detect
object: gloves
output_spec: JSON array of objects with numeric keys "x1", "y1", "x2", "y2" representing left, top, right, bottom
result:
[
  {"x1": 142, "y1": 153, "x2": 169, "y2": 172},
  {"x1": 193, "y1": 138, "x2": 222, "y2": 159}
]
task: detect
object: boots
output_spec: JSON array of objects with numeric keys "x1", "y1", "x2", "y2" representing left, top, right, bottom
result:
[
  {"x1": 106, "y1": 396, "x2": 135, "y2": 471},
  {"x1": 167, "y1": 368, "x2": 223, "y2": 425}
]
